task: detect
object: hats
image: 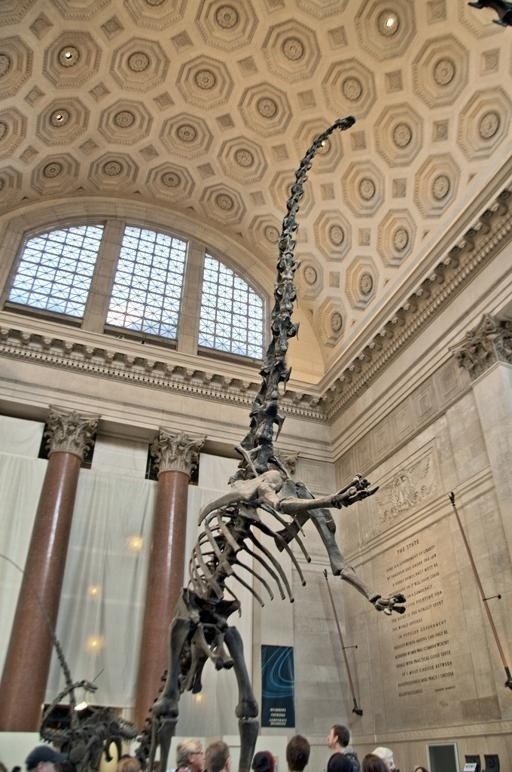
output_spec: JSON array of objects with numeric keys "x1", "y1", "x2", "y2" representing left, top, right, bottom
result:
[
  {"x1": 24, "y1": 744, "x2": 69, "y2": 766},
  {"x1": 370, "y1": 746, "x2": 395, "y2": 770}
]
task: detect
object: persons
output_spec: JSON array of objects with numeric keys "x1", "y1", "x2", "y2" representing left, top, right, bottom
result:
[{"x1": 0, "y1": 723, "x2": 429, "y2": 772}]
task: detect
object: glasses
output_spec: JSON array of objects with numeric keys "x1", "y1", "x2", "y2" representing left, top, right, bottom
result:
[{"x1": 190, "y1": 751, "x2": 204, "y2": 757}]
